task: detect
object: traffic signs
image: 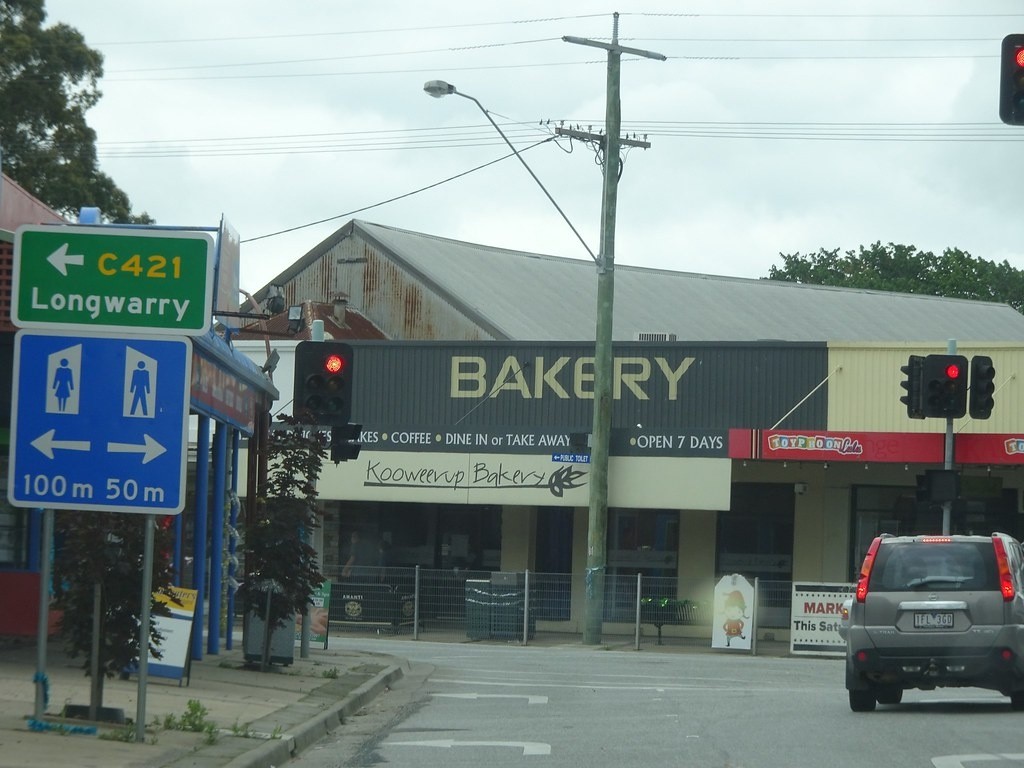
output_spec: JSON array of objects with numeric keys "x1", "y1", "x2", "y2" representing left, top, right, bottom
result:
[
  {"x1": 10, "y1": 221, "x2": 215, "y2": 335},
  {"x1": 8, "y1": 330, "x2": 191, "y2": 517}
]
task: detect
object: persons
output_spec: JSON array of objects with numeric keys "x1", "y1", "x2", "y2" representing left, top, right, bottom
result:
[
  {"x1": 343, "y1": 531, "x2": 369, "y2": 583},
  {"x1": 636, "y1": 545, "x2": 654, "y2": 576},
  {"x1": 377, "y1": 541, "x2": 395, "y2": 573}
]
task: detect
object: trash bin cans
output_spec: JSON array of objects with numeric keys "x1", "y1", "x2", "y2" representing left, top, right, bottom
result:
[
  {"x1": 242, "y1": 600, "x2": 296, "y2": 665},
  {"x1": 465, "y1": 580, "x2": 490, "y2": 640},
  {"x1": 490, "y1": 571, "x2": 536, "y2": 641}
]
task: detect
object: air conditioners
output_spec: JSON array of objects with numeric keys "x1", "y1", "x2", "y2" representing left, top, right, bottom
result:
[{"x1": 632, "y1": 331, "x2": 677, "y2": 342}]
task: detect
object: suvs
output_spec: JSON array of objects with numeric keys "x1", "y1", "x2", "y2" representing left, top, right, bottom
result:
[{"x1": 845, "y1": 531, "x2": 1024, "y2": 712}]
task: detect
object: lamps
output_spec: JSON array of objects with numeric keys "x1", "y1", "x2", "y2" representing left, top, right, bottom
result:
[
  {"x1": 261, "y1": 349, "x2": 281, "y2": 375},
  {"x1": 286, "y1": 305, "x2": 303, "y2": 320},
  {"x1": 987, "y1": 465, "x2": 990, "y2": 471},
  {"x1": 824, "y1": 461, "x2": 827, "y2": 468},
  {"x1": 905, "y1": 463, "x2": 908, "y2": 470},
  {"x1": 784, "y1": 461, "x2": 787, "y2": 468},
  {"x1": 864, "y1": 463, "x2": 868, "y2": 469},
  {"x1": 743, "y1": 459, "x2": 747, "y2": 466}
]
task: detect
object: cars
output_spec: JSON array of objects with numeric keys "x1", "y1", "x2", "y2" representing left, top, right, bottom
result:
[
  {"x1": 230, "y1": 579, "x2": 250, "y2": 615},
  {"x1": 839, "y1": 599, "x2": 854, "y2": 640}
]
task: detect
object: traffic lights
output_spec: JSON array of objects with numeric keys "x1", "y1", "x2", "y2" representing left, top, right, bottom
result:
[
  {"x1": 999, "y1": 34, "x2": 1024, "y2": 127},
  {"x1": 291, "y1": 340, "x2": 355, "y2": 425},
  {"x1": 901, "y1": 355, "x2": 927, "y2": 420},
  {"x1": 970, "y1": 355, "x2": 996, "y2": 420},
  {"x1": 924, "y1": 354, "x2": 969, "y2": 419},
  {"x1": 330, "y1": 422, "x2": 361, "y2": 464}
]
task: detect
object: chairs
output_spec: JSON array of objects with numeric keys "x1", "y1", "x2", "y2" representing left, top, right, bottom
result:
[{"x1": 900, "y1": 558, "x2": 928, "y2": 590}]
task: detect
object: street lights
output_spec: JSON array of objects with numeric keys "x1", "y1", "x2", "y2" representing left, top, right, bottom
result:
[{"x1": 425, "y1": 81, "x2": 615, "y2": 643}]
